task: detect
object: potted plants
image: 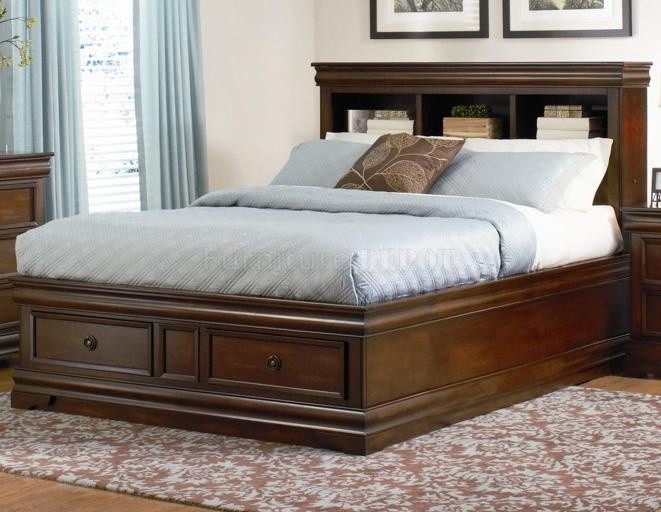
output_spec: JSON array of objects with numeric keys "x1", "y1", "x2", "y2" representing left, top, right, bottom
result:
[{"x1": 442, "y1": 103, "x2": 502, "y2": 139}]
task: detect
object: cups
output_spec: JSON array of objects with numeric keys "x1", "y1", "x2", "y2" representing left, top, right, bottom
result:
[{"x1": 348, "y1": 110, "x2": 372, "y2": 132}]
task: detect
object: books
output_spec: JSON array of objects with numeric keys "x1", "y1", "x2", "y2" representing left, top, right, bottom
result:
[
  {"x1": 536, "y1": 104, "x2": 604, "y2": 140},
  {"x1": 366, "y1": 110, "x2": 416, "y2": 135}
]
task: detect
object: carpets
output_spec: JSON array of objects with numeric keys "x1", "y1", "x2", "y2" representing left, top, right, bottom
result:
[{"x1": 0, "y1": 383, "x2": 661, "y2": 511}]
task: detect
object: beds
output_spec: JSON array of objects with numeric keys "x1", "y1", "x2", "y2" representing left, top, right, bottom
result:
[{"x1": 6, "y1": 60, "x2": 653, "y2": 456}]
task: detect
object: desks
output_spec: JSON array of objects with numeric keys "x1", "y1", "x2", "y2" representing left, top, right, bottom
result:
[{"x1": 0, "y1": 151, "x2": 55, "y2": 356}]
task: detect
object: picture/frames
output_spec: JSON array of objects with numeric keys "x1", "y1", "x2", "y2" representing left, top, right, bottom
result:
[
  {"x1": 502, "y1": 0, "x2": 632, "y2": 38},
  {"x1": 369, "y1": 0, "x2": 489, "y2": 39},
  {"x1": 652, "y1": 168, "x2": 661, "y2": 193}
]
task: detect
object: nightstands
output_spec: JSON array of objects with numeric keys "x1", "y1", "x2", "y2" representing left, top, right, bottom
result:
[{"x1": 619, "y1": 206, "x2": 661, "y2": 378}]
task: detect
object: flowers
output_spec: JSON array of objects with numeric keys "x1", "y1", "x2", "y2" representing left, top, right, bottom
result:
[{"x1": 0, "y1": 0, "x2": 37, "y2": 68}]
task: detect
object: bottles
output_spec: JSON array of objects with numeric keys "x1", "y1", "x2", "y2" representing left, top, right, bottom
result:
[{"x1": 374, "y1": 110, "x2": 410, "y2": 120}]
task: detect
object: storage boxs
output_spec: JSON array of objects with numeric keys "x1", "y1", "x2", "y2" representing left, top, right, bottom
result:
[
  {"x1": 366, "y1": 118, "x2": 415, "y2": 136},
  {"x1": 374, "y1": 109, "x2": 411, "y2": 120},
  {"x1": 543, "y1": 105, "x2": 592, "y2": 118},
  {"x1": 536, "y1": 116, "x2": 604, "y2": 139}
]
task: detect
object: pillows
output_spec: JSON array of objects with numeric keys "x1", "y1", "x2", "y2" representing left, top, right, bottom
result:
[
  {"x1": 427, "y1": 146, "x2": 596, "y2": 214},
  {"x1": 269, "y1": 138, "x2": 373, "y2": 188},
  {"x1": 465, "y1": 137, "x2": 614, "y2": 211},
  {"x1": 325, "y1": 131, "x2": 465, "y2": 145},
  {"x1": 335, "y1": 132, "x2": 465, "y2": 193}
]
